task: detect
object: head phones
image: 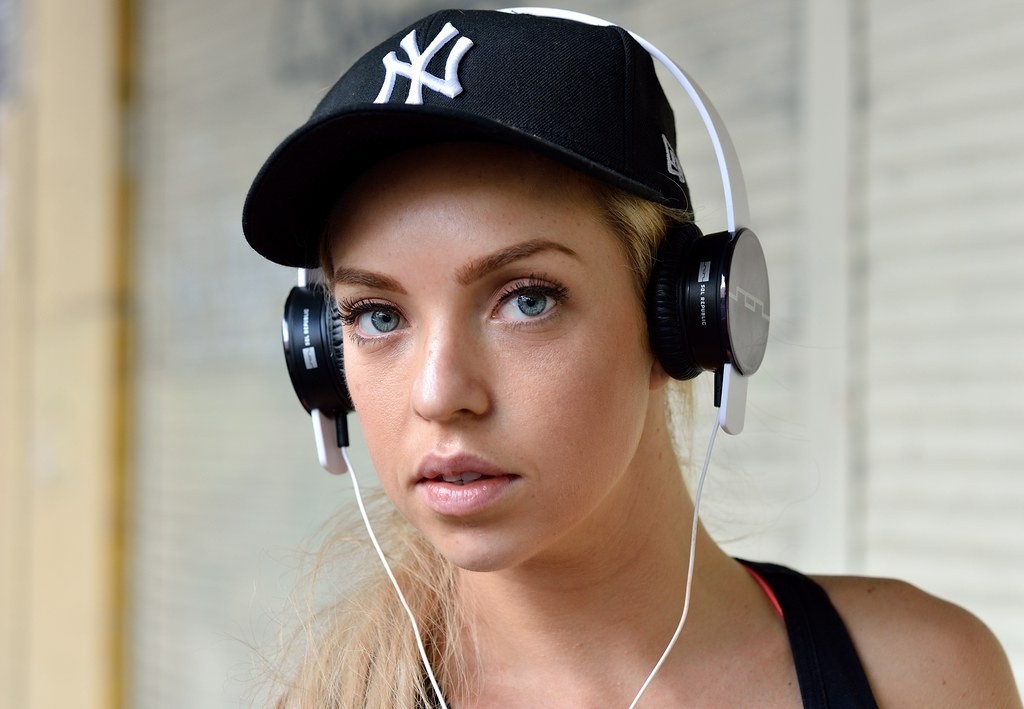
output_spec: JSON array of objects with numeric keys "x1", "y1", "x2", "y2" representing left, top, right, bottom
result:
[{"x1": 282, "y1": 6, "x2": 771, "y2": 475}]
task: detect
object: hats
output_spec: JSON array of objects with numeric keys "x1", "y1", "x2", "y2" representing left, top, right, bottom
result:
[{"x1": 241, "y1": 9, "x2": 696, "y2": 269}]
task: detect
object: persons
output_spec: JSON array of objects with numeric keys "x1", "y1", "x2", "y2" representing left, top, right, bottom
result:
[{"x1": 242, "y1": 11, "x2": 1019, "y2": 709}]
